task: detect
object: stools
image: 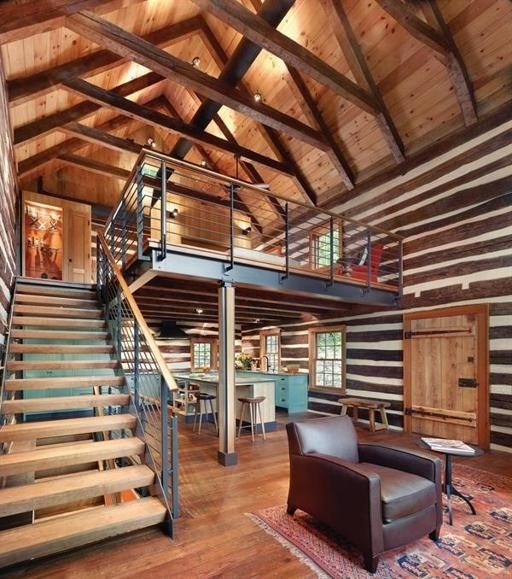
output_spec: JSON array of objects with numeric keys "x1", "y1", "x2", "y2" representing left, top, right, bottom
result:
[
  {"x1": 193, "y1": 392, "x2": 219, "y2": 436},
  {"x1": 337, "y1": 257, "x2": 358, "y2": 279},
  {"x1": 238, "y1": 397, "x2": 267, "y2": 442}
]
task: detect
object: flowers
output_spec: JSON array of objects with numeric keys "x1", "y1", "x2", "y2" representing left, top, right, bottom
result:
[{"x1": 236, "y1": 352, "x2": 253, "y2": 367}]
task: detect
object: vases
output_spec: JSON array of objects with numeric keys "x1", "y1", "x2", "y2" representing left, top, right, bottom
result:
[{"x1": 244, "y1": 364, "x2": 249, "y2": 370}]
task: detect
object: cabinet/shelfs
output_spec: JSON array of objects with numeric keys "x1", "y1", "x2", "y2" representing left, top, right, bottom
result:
[
  {"x1": 275, "y1": 376, "x2": 308, "y2": 415},
  {"x1": 172, "y1": 380, "x2": 201, "y2": 415}
]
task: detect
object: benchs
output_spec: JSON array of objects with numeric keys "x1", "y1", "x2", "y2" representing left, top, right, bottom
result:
[{"x1": 338, "y1": 398, "x2": 391, "y2": 433}]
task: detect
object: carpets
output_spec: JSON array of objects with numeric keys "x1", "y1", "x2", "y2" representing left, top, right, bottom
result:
[{"x1": 243, "y1": 462, "x2": 512, "y2": 579}]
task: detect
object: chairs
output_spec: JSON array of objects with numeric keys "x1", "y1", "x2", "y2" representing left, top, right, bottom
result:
[
  {"x1": 284, "y1": 414, "x2": 443, "y2": 574},
  {"x1": 336, "y1": 242, "x2": 383, "y2": 283}
]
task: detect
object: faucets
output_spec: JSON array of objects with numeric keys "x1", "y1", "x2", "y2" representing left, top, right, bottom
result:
[{"x1": 259, "y1": 355, "x2": 271, "y2": 371}]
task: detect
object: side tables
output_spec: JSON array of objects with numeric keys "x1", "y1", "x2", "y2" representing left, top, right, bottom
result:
[{"x1": 414, "y1": 437, "x2": 485, "y2": 525}]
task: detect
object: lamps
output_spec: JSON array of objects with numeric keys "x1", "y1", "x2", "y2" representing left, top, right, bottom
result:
[
  {"x1": 169, "y1": 208, "x2": 178, "y2": 218},
  {"x1": 253, "y1": 89, "x2": 261, "y2": 102},
  {"x1": 201, "y1": 160, "x2": 207, "y2": 167},
  {"x1": 147, "y1": 137, "x2": 156, "y2": 148},
  {"x1": 191, "y1": 56, "x2": 200, "y2": 67},
  {"x1": 242, "y1": 226, "x2": 252, "y2": 235}
]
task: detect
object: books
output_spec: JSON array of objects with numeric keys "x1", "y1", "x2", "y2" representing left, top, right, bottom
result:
[{"x1": 421, "y1": 437, "x2": 477, "y2": 453}]
